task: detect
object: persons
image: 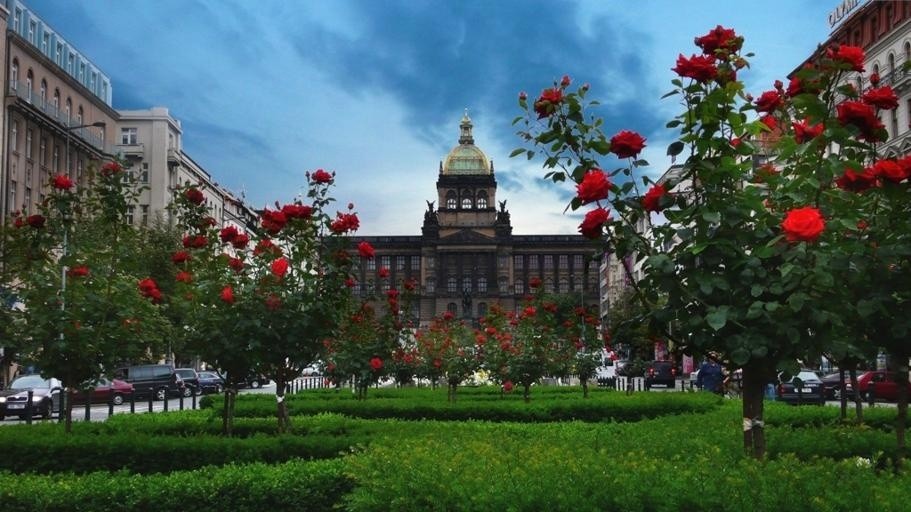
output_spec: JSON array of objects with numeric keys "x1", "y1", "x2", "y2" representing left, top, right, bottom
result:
[
  {"x1": 726, "y1": 367, "x2": 743, "y2": 400},
  {"x1": 697, "y1": 351, "x2": 726, "y2": 398}
]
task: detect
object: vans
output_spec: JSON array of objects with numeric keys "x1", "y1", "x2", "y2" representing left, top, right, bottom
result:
[{"x1": 101, "y1": 365, "x2": 175, "y2": 400}]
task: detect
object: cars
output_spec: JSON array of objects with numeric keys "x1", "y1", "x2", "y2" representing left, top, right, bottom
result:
[
  {"x1": 1, "y1": 372, "x2": 73, "y2": 419},
  {"x1": 293, "y1": 358, "x2": 328, "y2": 377},
  {"x1": 774, "y1": 367, "x2": 826, "y2": 407},
  {"x1": 174, "y1": 366, "x2": 271, "y2": 398},
  {"x1": 690, "y1": 361, "x2": 824, "y2": 394},
  {"x1": 69, "y1": 371, "x2": 136, "y2": 406},
  {"x1": 563, "y1": 347, "x2": 645, "y2": 378},
  {"x1": 819, "y1": 368, "x2": 881, "y2": 400},
  {"x1": 643, "y1": 360, "x2": 676, "y2": 388},
  {"x1": 845, "y1": 370, "x2": 911, "y2": 404}
]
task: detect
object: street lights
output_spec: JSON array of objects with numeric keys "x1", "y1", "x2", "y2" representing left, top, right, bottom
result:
[
  {"x1": 60, "y1": 120, "x2": 106, "y2": 365},
  {"x1": 220, "y1": 213, "x2": 247, "y2": 258},
  {"x1": 569, "y1": 273, "x2": 584, "y2": 329}
]
